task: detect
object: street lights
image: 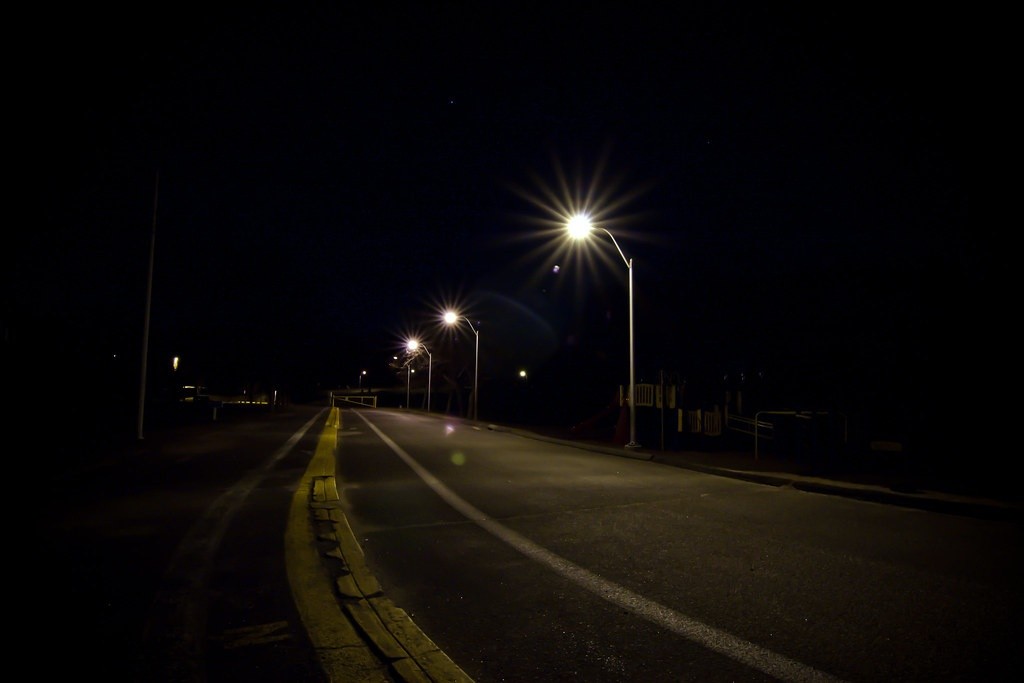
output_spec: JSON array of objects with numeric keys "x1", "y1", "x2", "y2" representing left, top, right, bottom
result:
[
  {"x1": 393, "y1": 355, "x2": 410, "y2": 412},
  {"x1": 407, "y1": 339, "x2": 432, "y2": 413},
  {"x1": 359, "y1": 371, "x2": 367, "y2": 388},
  {"x1": 444, "y1": 311, "x2": 479, "y2": 426},
  {"x1": 565, "y1": 215, "x2": 640, "y2": 452}
]
